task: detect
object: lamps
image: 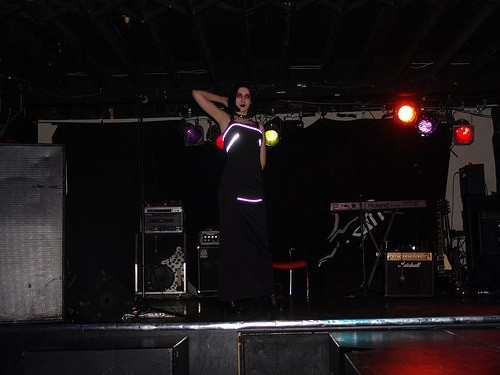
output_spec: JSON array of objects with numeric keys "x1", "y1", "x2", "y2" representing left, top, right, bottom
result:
[
  {"x1": 265, "y1": 124, "x2": 281, "y2": 149},
  {"x1": 184, "y1": 125, "x2": 203, "y2": 143},
  {"x1": 452, "y1": 118, "x2": 474, "y2": 145},
  {"x1": 206, "y1": 125, "x2": 221, "y2": 143}
]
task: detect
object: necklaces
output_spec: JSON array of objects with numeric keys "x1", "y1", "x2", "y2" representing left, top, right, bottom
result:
[{"x1": 235, "y1": 112, "x2": 249, "y2": 118}]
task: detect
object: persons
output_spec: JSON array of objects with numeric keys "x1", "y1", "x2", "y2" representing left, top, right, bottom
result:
[{"x1": 192, "y1": 83, "x2": 288, "y2": 314}]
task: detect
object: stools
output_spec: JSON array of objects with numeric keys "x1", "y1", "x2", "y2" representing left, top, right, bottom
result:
[{"x1": 271, "y1": 256, "x2": 309, "y2": 304}]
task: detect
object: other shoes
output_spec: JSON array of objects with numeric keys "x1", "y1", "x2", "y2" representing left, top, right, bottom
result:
[
  {"x1": 265, "y1": 293, "x2": 288, "y2": 315},
  {"x1": 227, "y1": 298, "x2": 244, "y2": 316}
]
